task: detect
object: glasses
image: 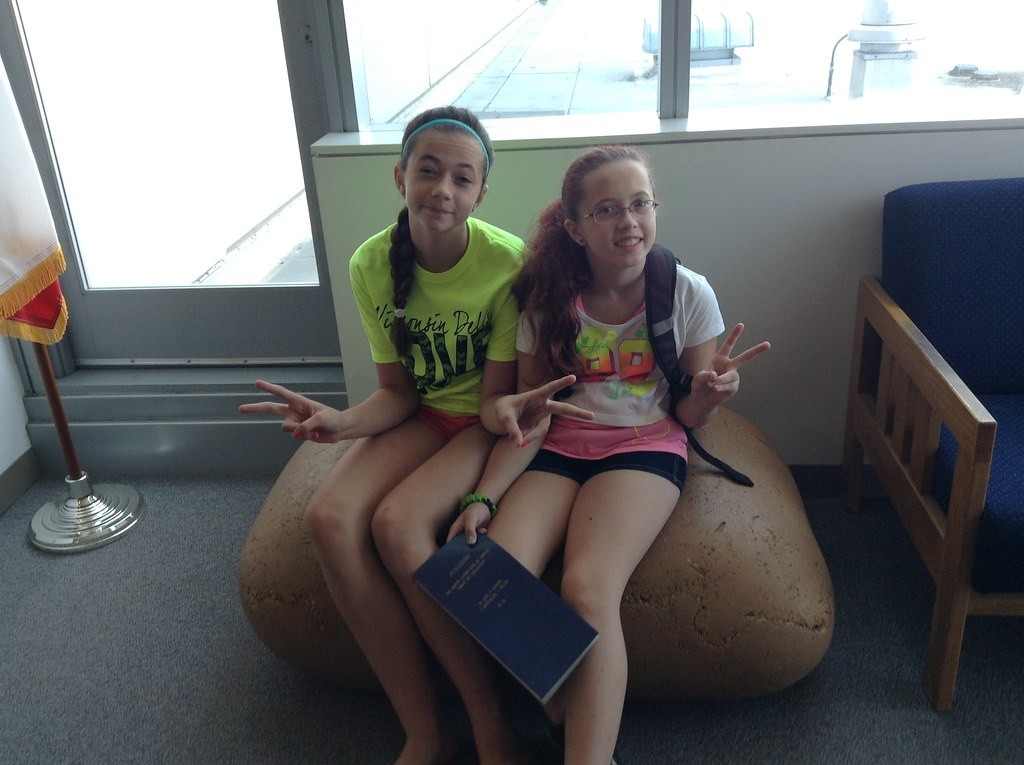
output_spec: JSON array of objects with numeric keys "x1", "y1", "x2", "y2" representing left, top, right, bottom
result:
[{"x1": 576, "y1": 200, "x2": 660, "y2": 224}]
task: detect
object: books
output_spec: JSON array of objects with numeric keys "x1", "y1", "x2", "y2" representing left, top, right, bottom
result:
[{"x1": 412, "y1": 527, "x2": 601, "y2": 705}]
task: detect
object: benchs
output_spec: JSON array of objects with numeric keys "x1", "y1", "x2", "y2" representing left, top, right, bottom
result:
[{"x1": 238, "y1": 401, "x2": 834, "y2": 702}]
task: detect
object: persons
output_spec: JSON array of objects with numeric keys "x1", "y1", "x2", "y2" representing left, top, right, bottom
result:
[
  {"x1": 239, "y1": 107, "x2": 595, "y2": 765},
  {"x1": 447, "y1": 147, "x2": 771, "y2": 765}
]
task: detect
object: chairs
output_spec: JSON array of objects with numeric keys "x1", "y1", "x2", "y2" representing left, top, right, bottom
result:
[{"x1": 837, "y1": 175, "x2": 1024, "y2": 711}]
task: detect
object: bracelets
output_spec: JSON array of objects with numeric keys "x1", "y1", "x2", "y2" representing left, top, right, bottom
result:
[{"x1": 459, "y1": 493, "x2": 499, "y2": 519}]
